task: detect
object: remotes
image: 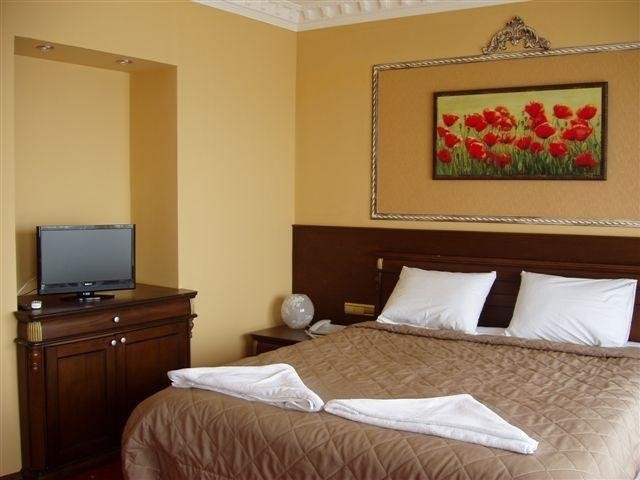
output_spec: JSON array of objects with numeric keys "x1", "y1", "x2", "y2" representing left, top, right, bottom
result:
[{"x1": 31, "y1": 301, "x2": 42, "y2": 309}]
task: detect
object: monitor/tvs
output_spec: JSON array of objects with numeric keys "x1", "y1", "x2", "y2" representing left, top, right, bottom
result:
[{"x1": 35, "y1": 224, "x2": 137, "y2": 302}]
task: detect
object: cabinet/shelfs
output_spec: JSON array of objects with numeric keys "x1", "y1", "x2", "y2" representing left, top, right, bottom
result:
[{"x1": 13, "y1": 282, "x2": 199, "y2": 480}]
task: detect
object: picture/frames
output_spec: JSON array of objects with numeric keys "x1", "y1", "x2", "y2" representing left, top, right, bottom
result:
[{"x1": 371, "y1": 14, "x2": 640, "y2": 228}]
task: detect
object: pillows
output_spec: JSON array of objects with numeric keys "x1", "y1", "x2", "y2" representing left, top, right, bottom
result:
[
  {"x1": 375, "y1": 265, "x2": 496, "y2": 335},
  {"x1": 501, "y1": 270, "x2": 638, "y2": 347}
]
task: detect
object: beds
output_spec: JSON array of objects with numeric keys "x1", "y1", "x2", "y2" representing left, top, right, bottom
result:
[{"x1": 120, "y1": 251, "x2": 640, "y2": 480}]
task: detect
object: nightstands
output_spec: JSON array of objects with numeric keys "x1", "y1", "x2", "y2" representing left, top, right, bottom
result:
[{"x1": 250, "y1": 327, "x2": 311, "y2": 355}]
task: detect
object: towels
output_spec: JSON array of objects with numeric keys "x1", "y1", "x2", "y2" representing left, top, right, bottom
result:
[
  {"x1": 166, "y1": 362, "x2": 325, "y2": 413},
  {"x1": 322, "y1": 392, "x2": 539, "y2": 456}
]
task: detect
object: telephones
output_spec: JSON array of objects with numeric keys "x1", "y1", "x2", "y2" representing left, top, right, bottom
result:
[{"x1": 308, "y1": 319, "x2": 348, "y2": 335}]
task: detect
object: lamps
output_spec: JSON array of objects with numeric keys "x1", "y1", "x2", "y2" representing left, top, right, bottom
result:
[{"x1": 280, "y1": 294, "x2": 315, "y2": 329}]
